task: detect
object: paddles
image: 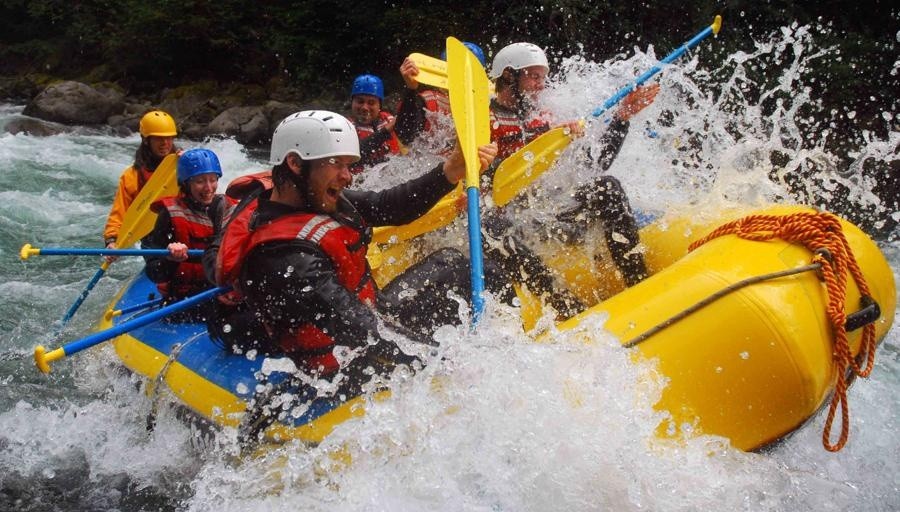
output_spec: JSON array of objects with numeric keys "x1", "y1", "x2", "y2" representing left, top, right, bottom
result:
[
  {"x1": 37, "y1": 181, "x2": 467, "y2": 373},
  {"x1": 54, "y1": 152, "x2": 180, "y2": 330},
  {"x1": 410, "y1": 52, "x2": 448, "y2": 89},
  {"x1": 492, "y1": 10, "x2": 721, "y2": 206},
  {"x1": 447, "y1": 36, "x2": 492, "y2": 328}
]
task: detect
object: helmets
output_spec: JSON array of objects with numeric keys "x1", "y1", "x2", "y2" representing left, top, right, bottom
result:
[
  {"x1": 351, "y1": 74, "x2": 384, "y2": 102},
  {"x1": 139, "y1": 110, "x2": 178, "y2": 137},
  {"x1": 489, "y1": 42, "x2": 550, "y2": 80},
  {"x1": 268, "y1": 110, "x2": 362, "y2": 166},
  {"x1": 441, "y1": 42, "x2": 486, "y2": 66},
  {"x1": 177, "y1": 149, "x2": 222, "y2": 185}
]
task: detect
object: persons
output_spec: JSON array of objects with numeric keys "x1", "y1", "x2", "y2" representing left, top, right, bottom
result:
[
  {"x1": 341, "y1": 74, "x2": 402, "y2": 176},
  {"x1": 140, "y1": 149, "x2": 238, "y2": 323},
  {"x1": 103, "y1": 110, "x2": 184, "y2": 262},
  {"x1": 205, "y1": 109, "x2": 497, "y2": 380},
  {"x1": 393, "y1": 43, "x2": 485, "y2": 161},
  {"x1": 457, "y1": 40, "x2": 650, "y2": 320}
]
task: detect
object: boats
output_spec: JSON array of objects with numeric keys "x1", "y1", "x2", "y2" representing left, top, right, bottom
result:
[{"x1": 96, "y1": 200, "x2": 898, "y2": 493}]
